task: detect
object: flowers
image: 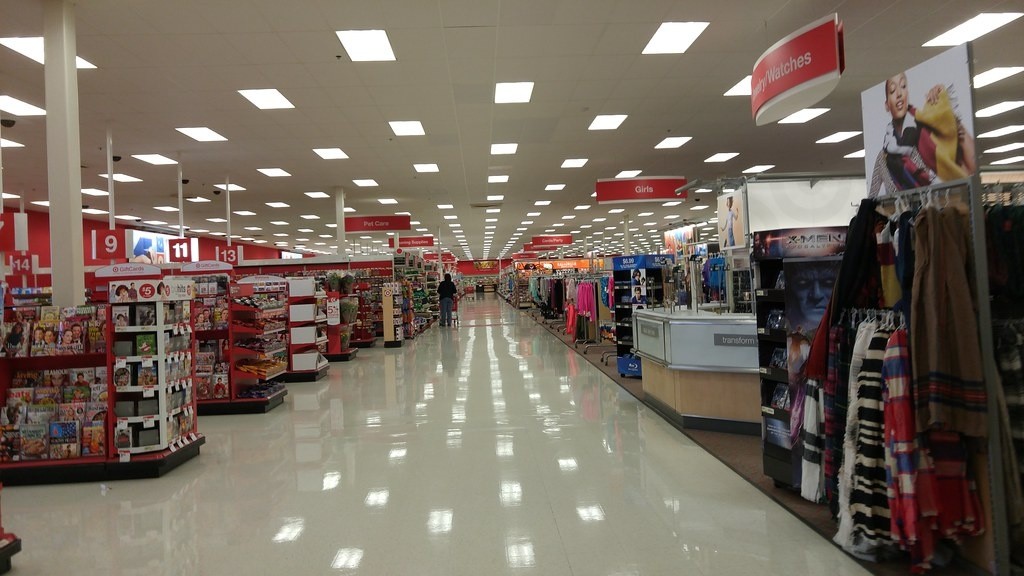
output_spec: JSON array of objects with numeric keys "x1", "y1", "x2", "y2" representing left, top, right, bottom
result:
[
  {"x1": 328, "y1": 273, "x2": 341, "y2": 283},
  {"x1": 340, "y1": 331, "x2": 348, "y2": 342}
]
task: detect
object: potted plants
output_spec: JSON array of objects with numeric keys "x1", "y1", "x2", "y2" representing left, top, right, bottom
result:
[
  {"x1": 340, "y1": 274, "x2": 353, "y2": 294},
  {"x1": 352, "y1": 301, "x2": 358, "y2": 324},
  {"x1": 340, "y1": 302, "x2": 350, "y2": 324},
  {"x1": 348, "y1": 303, "x2": 354, "y2": 323}
]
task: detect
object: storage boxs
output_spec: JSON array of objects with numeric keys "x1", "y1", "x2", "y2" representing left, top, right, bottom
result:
[
  {"x1": 137, "y1": 335, "x2": 156, "y2": 356},
  {"x1": 20, "y1": 423, "x2": 49, "y2": 461},
  {"x1": 0, "y1": 425, "x2": 19, "y2": 462},
  {"x1": 114, "y1": 365, "x2": 132, "y2": 386},
  {"x1": 163, "y1": 300, "x2": 190, "y2": 325},
  {"x1": 1, "y1": 304, "x2": 107, "y2": 424},
  {"x1": 81, "y1": 420, "x2": 104, "y2": 457},
  {"x1": 115, "y1": 401, "x2": 135, "y2": 418},
  {"x1": 166, "y1": 354, "x2": 192, "y2": 383},
  {"x1": 137, "y1": 400, "x2": 159, "y2": 416},
  {"x1": 114, "y1": 341, "x2": 133, "y2": 357},
  {"x1": 167, "y1": 410, "x2": 194, "y2": 441},
  {"x1": 137, "y1": 429, "x2": 159, "y2": 445},
  {"x1": 166, "y1": 384, "x2": 192, "y2": 411},
  {"x1": 112, "y1": 306, "x2": 130, "y2": 327},
  {"x1": 164, "y1": 330, "x2": 190, "y2": 353},
  {"x1": 48, "y1": 420, "x2": 81, "y2": 459},
  {"x1": 138, "y1": 365, "x2": 158, "y2": 386},
  {"x1": 114, "y1": 427, "x2": 133, "y2": 449},
  {"x1": 136, "y1": 305, "x2": 156, "y2": 326}
]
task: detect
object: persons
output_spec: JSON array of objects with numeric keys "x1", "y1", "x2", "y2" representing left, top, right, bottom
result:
[
  {"x1": 0, "y1": 320, "x2": 106, "y2": 360},
  {"x1": 1, "y1": 372, "x2": 108, "y2": 431},
  {"x1": 134, "y1": 237, "x2": 157, "y2": 266},
  {"x1": 113, "y1": 311, "x2": 129, "y2": 326},
  {"x1": 869, "y1": 73, "x2": 975, "y2": 199},
  {"x1": 437, "y1": 273, "x2": 457, "y2": 327},
  {"x1": 195, "y1": 295, "x2": 230, "y2": 399},
  {"x1": 631, "y1": 269, "x2": 645, "y2": 285},
  {"x1": 110, "y1": 281, "x2": 168, "y2": 303},
  {"x1": 632, "y1": 287, "x2": 647, "y2": 304},
  {"x1": 719, "y1": 196, "x2": 739, "y2": 247}
]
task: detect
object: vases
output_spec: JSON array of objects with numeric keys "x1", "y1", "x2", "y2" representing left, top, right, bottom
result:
[
  {"x1": 330, "y1": 281, "x2": 340, "y2": 293},
  {"x1": 341, "y1": 341, "x2": 345, "y2": 352}
]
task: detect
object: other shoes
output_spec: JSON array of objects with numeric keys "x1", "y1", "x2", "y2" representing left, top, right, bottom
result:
[
  {"x1": 439, "y1": 322, "x2": 445, "y2": 327},
  {"x1": 447, "y1": 323, "x2": 451, "y2": 326}
]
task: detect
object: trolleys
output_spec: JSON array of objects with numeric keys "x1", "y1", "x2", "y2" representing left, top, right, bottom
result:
[
  {"x1": 438, "y1": 295, "x2": 458, "y2": 326},
  {"x1": 464, "y1": 286, "x2": 474, "y2": 301}
]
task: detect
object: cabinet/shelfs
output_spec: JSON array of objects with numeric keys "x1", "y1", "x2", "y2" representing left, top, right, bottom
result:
[
  {"x1": 275, "y1": 277, "x2": 331, "y2": 386},
  {"x1": 350, "y1": 279, "x2": 377, "y2": 349},
  {"x1": 232, "y1": 254, "x2": 419, "y2": 340},
  {"x1": 317, "y1": 271, "x2": 362, "y2": 362},
  {"x1": 195, "y1": 272, "x2": 289, "y2": 416},
  {"x1": 0, "y1": 279, "x2": 206, "y2": 486},
  {"x1": 410, "y1": 252, "x2": 463, "y2": 333}
]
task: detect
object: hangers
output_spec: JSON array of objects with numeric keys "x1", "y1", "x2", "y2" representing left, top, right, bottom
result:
[
  {"x1": 891, "y1": 187, "x2": 952, "y2": 213},
  {"x1": 708, "y1": 252, "x2": 726, "y2": 261},
  {"x1": 531, "y1": 273, "x2": 598, "y2": 285},
  {"x1": 838, "y1": 305, "x2": 907, "y2": 334}
]
